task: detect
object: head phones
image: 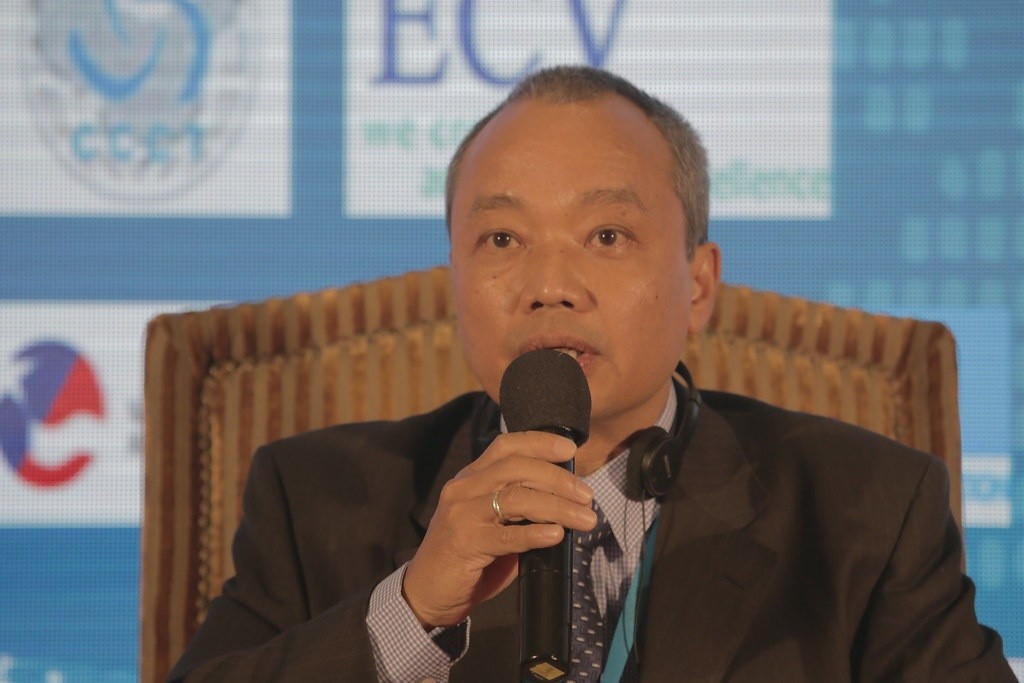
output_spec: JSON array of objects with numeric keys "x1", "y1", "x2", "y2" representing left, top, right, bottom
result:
[{"x1": 472, "y1": 357, "x2": 700, "y2": 501}]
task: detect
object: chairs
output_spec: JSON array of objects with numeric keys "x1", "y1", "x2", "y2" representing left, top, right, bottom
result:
[{"x1": 141, "y1": 267, "x2": 962, "y2": 683}]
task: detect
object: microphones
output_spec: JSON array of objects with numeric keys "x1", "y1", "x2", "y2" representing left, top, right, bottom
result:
[{"x1": 499, "y1": 349, "x2": 592, "y2": 683}]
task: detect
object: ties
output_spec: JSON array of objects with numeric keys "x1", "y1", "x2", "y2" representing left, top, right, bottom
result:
[{"x1": 568, "y1": 500, "x2": 612, "y2": 683}]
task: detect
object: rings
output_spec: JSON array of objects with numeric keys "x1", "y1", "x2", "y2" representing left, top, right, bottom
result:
[{"x1": 492, "y1": 489, "x2": 505, "y2": 521}]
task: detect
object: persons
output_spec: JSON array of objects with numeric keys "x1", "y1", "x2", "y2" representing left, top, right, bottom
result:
[{"x1": 162, "y1": 65, "x2": 1018, "y2": 683}]
